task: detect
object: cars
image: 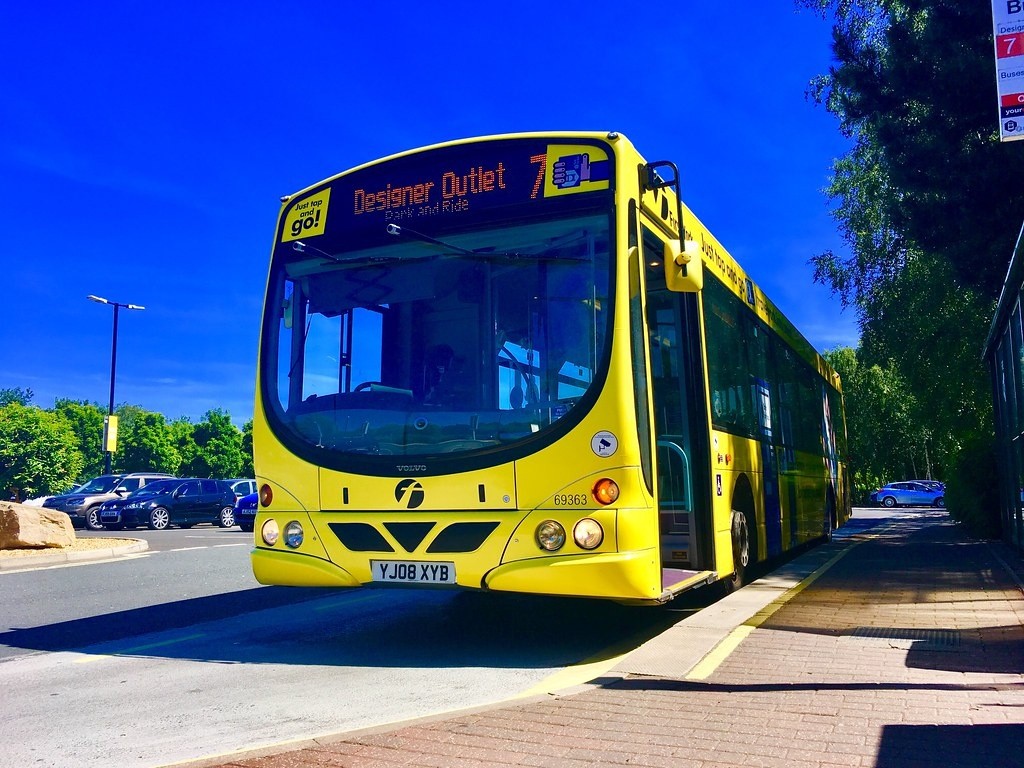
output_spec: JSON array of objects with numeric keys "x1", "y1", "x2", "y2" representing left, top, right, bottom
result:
[
  {"x1": 96, "y1": 478, "x2": 239, "y2": 531},
  {"x1": 21, "y1": 482, "x2": 83, "y2": 507},
  {"x1": 870, "y1": 481, "x2": 946, "y2": 509},
  {"x1": 219, "y1": 478, "x2": 258, "y2": 507},
  {"x1": 233, "y1": 492, "x2": 260, "y2": 533}
]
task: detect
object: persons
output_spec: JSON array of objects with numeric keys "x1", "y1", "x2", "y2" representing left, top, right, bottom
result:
[{"x1": 422, "y1": 343, "x2": 487, "y2": 406}]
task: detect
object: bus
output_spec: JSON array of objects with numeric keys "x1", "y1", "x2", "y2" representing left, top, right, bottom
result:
[{"x1": 252, "y1": 131, "x2": 854, "y2": 607}]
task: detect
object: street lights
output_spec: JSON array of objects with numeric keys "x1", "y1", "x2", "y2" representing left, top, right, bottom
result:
[{"x1": 86, "y1": 294, "x2": 145, "y2": 476}]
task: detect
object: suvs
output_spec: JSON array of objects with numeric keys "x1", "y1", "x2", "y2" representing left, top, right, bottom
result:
[{"x1": 42, "y1": 472, "x2": 185, "y2": 530}]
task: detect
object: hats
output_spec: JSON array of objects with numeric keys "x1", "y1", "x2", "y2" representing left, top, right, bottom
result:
[{"x1": 428, "y1": 344, "x2": 454, "y2": 362}]
